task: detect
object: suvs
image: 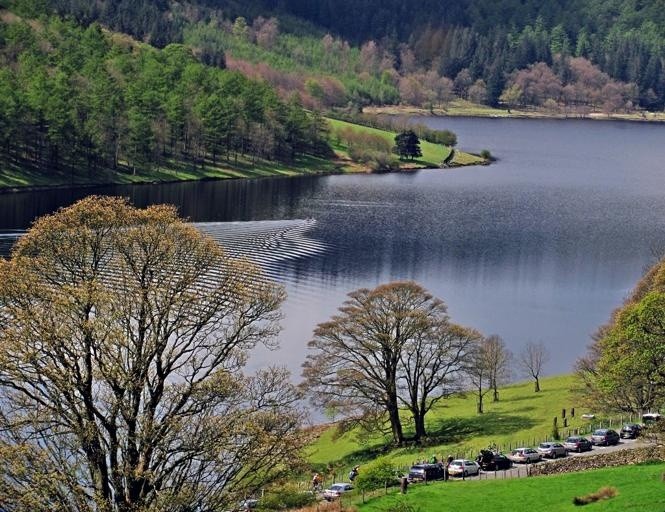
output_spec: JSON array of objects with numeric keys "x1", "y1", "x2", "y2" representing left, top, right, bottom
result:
[{"x1": 407, "y1": 413, "x2": 663, "y2": 485}]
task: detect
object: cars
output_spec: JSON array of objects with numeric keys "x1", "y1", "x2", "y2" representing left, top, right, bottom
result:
[
  {"x1": 240, "y1": 498, "x2": 262, "y2": 512},
  {"x1": 323, "y1": 482, "x2": 360, "y2": 501}
]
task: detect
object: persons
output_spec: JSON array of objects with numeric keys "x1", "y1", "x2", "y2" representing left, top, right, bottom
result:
[
  {"x1": 312, "y1": 473, "x2": 319, "y2": 487},
  {"x1": 350, "y1": 467, "x2": 359, "y2": 482},
  {"x1": 447, "y1": 455, "x2": 453, "y2": 466},
  {"x1": 475, "y1": 450, "x2": 494, "y2": 463},
  {"x1": 432, "y1": 455, "x2": 438, "y2": 464}
]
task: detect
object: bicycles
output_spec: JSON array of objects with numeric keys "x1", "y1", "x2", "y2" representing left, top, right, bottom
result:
[{"x1": 310, "y1": 479, "x2": 325, "y2": 495}]
